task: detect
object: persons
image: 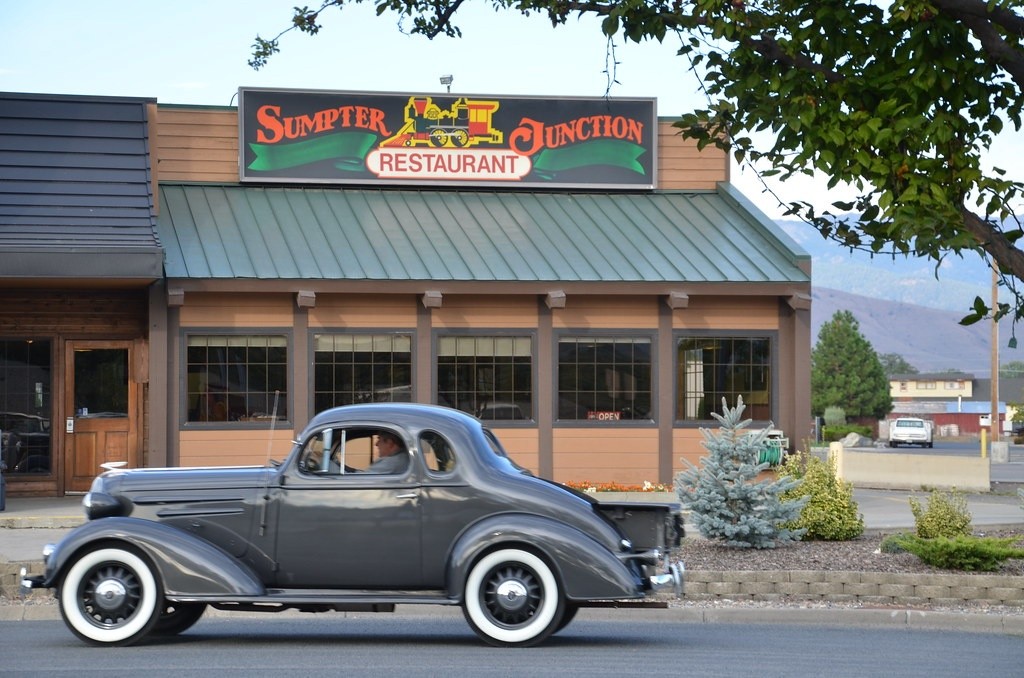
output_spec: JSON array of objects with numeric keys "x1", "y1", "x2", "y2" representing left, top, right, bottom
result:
[
  {"x1": 312, "y1": 431, "x2": 410, "y2": 474},
  {"x1": 199, "y1": 390, "x2": 226, "y2": 422}
]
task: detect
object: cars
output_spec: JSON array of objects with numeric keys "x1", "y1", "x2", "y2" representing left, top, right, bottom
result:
[
  {"x1": 0, "y1": 412, "x2": 53, "y2": 473},
  {"x1": 478, "y1": 401, "x2": 524, "y2": 420},
  {"x1": 18, "y1": 401, "x2": 690, "y2": 647},
  {"x1": 890, "y1": 418, "x2": 934, "y2": 448}
]
